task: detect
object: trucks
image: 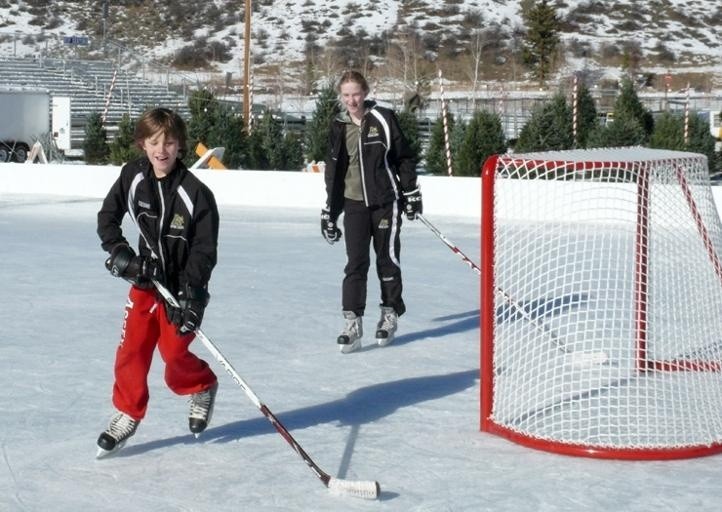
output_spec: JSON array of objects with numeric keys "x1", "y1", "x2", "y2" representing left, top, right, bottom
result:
[{"x1": 0, "y1": 89, "x2": 52, "y2": 162}]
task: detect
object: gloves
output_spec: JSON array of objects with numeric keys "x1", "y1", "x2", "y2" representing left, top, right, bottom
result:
[
  {"x1": 401, "y1": 185, "x2": 422, "y2": 220},
  {"x1": 321, "y1": 207, "x2": 342, "y2": 246},
  {"x1": 165, "y1": 283, "x2": 210, "y2": 337},
  {"x1": 104, "y1": 247, "x2": 162, "y2": 290}
]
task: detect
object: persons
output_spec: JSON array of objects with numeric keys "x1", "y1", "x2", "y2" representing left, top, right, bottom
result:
[
  {"x1": 321, "y1": 71, "x2": 421, "y2": 354},
  {"x1": 98, "y1": 108, "x2": 219, "y2": 460}
]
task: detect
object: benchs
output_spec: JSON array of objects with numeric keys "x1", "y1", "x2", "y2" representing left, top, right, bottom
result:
[{"x1": 1, "y1": 57, "x2": 197, "y2": 146}]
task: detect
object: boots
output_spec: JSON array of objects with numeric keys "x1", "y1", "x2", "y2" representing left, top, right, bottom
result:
[
  {"x1": 375, "y1": 304, "x2": 398, "y2": 338},
  {"x1": 337, "y1": 310, "x2": 363, "y2": 345}
]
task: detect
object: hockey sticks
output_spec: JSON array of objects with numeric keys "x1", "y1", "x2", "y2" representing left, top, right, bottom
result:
[
  {"x1": 147, "y1": 275, "x2": 380, "y2": 501},
  {"x1": 416, "y1": 214, "x2": 607, "y2": 364}
]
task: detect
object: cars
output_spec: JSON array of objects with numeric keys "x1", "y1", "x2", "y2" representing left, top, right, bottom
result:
[{"x1": 540, "y1": 67, "x2": 656, "y2": 95}]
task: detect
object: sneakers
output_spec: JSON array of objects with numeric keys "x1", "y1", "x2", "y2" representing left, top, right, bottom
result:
[
  {"x1": 96, "y1": 412, "x2": 140, "y2": 450},
  {"x1": 188, "y1": 382, "x2": 218, "y2": 432}
]
